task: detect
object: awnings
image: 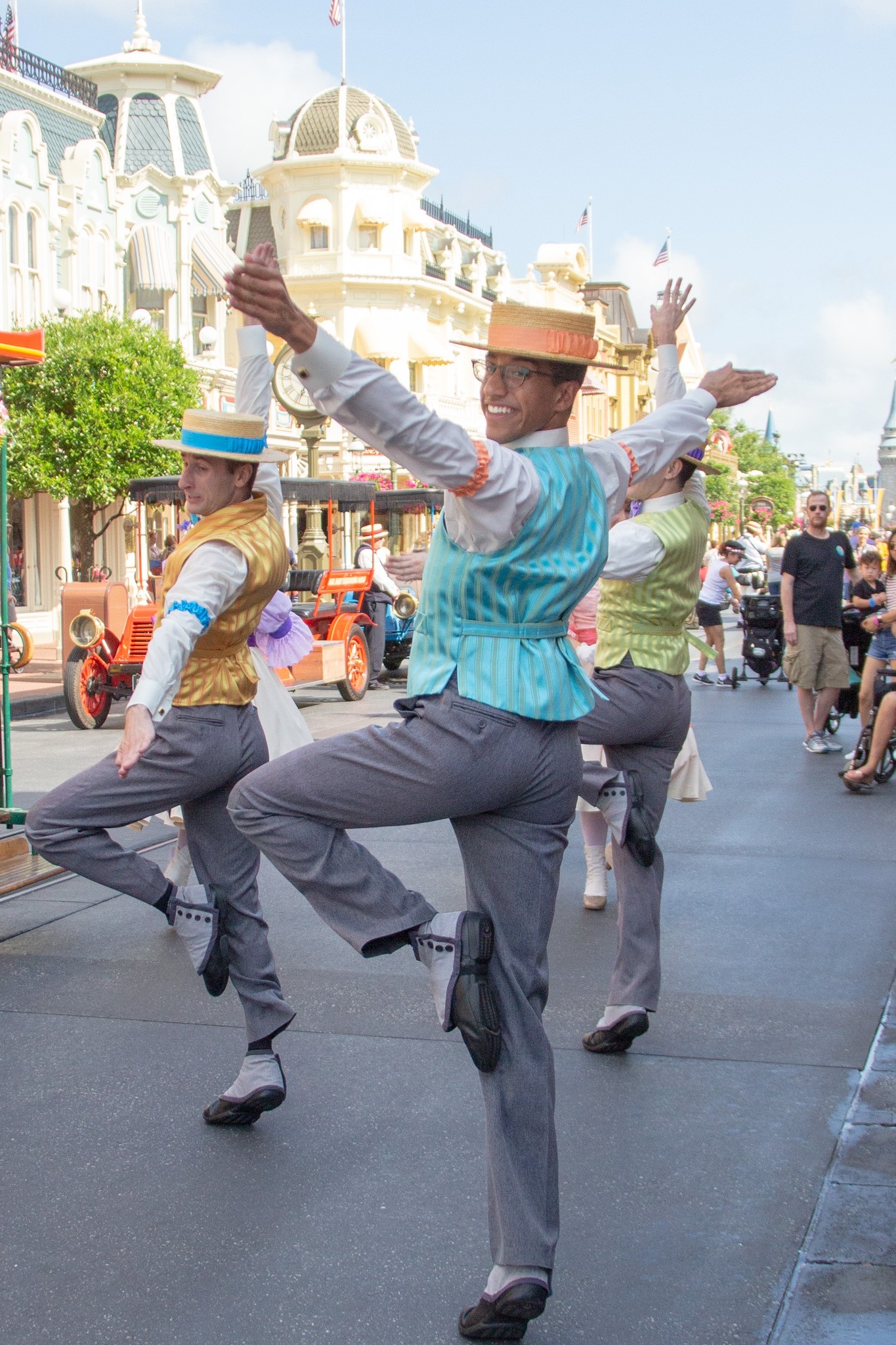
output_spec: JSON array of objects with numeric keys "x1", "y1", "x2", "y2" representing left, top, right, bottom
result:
[
  {"x1": 408, "y1": 326, "x2": 455, "y2": 367},
  {"x1": 356, "y1": 196, "x2": 389, "y2": 230},
  {"x1": 580, "y1": 371, "x2": 607, "y2": 397},
  {"x1": 191, "y1": 230, "x2": 245, "y2": 301},
  {"x1": 355, "y1": 322, "x2": 400, "y2": 365},
  {"x1": 313, "y1": 316, "x2": 337, "y2": 339},
  {"x1": 129, "y1": 226, "x2": 178, "y2": 295},
  {"x1": 296, "y1": 194, "x2": 332, "y2": 228},
  {"x1": 402, "y1": 199, "x2": 435, "y2": 232}
]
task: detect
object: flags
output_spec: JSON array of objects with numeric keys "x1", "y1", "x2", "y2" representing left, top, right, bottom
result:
[
  {"x1": 577, "y1": 206, "x2": 588, "y2": 230},
  {"x1": 329, "y1": 0, "x2": 341, "y2": 27},
  {"x1": 652, "y1": 240, "x2": 669, "y2": 267},
  {"x1": 0, "y1": 0, "x2": 19, "y2": 74}
]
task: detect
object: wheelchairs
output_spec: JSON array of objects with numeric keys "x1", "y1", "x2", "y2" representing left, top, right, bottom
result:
[
  {"x1": 824, "y1": 602, "x2": 886, "y2": 735},
  {"x1": 836, "y1": 666, "x2": 896, "y2": 795}
]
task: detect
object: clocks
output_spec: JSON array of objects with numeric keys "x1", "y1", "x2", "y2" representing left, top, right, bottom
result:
[{"x1": 272, "y1": 344, "x2": 326, "y2": 420}]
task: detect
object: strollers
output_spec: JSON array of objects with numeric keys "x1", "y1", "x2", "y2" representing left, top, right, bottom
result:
[{"x1": 728, "y1": 595, "x2": 794, "y2": 690}]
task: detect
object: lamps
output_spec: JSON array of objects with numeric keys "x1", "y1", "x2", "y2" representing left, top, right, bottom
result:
[
  {"x1": 198, "y1": 326, "x2": 217, "y2": 359},
  {"x1": 132, "y1": 309, "x2": 152, "y2": 328},
  {"x1": 52, "y1": 287, "x2": 70, "y2": 318}
]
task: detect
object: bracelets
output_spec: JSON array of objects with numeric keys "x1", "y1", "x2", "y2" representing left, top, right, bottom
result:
[
  {"x1": 869, "y1": 598, "x2": 875, "y2": 608},
  {"x1": 877, "y1": 615, "x2": 883, "y2": 625},
  {"x1": 873, "y1": 615, "x2": 879, "y2": 626}
]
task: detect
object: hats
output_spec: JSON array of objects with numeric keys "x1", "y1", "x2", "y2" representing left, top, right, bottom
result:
[
  {"x1": 358, "y1": 522, "x2": 390, "y2": 539},
  {"x1": 724, "y1": 546, "x2": 749, "y2": 560},
  {"x1": 450, "y1": 300, "x2": 632, "y2": 372},
  {"x1": 152, "y1": 408, "x2": 290, "y2": 464}
]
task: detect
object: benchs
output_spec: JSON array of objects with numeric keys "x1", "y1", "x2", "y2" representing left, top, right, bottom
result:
[{"x1": 287, "y1": 568, "x2": 358, "y2": 618}]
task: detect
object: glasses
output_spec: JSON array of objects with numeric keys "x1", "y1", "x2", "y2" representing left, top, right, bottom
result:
[
  {"x1": 808, "y1": 503, "x2": 827, "y2": 512},
  {"x1": 469, "y1": 358, "x2": 562, "y2": 389},
  {"x1": 888, "y1": 542, "x2": 895, "y2": 550}
]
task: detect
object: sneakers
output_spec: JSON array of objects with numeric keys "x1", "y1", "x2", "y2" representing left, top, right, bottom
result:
[
  {"x1": 717, "y1": 676, "x2": 741, "y2": 686},
  {"x1": 692, "y1": 673, "x2": 715, "y2": 685},
  {"x1": 802, "y1": 731, "x2": 843, "y2": 752}
]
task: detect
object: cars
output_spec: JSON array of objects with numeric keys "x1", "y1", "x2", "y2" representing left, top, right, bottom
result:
[{"x1": 62, "y1": 476, "x2": 446, "y2": 729}]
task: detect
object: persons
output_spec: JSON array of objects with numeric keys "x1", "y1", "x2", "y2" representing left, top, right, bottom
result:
[
  {"x1": 5, "y1": 521, "x2": 16, "y2": 664},
  {"x1": 825, "y1": 523, "x2": 896, "y2": 787},
  {"x1": 25, "y1": 242, "x2": 285, "y2": 1130},
  {"x1": 384, "y1": 276, "x2": 722, "y2": 1055},
  {"x1": 354, "y1": 524, "x2": 400, "y2": 690},
  {"x1": 226, "y1": 252, "x2": 778, "y2": 1340},
  {"x1": 692, "y1": 521, "x2": 806, "y2": 687},
  {"x1": 283, "y1": 547, "x2": 299, "y2": 604},
  {"x1": 126, "y1": 589, "x2": 315, "y2": 903},
  {"x1": 568, "y1": 496, "x2": 712, "y2": 909},
  {"x1": 149, "y1": 532, "x2": 179, "y2": 576},
  {"x1": 780, "y1": 490, "x2": 860, "y2": 754}
]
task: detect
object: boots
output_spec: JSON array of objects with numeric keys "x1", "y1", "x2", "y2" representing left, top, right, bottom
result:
[{"x1": 579, "y1": 844, "x2": 609, "y2": 912}]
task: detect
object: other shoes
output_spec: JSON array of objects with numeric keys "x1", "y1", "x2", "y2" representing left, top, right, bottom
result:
[
  {"x1": 582, "y1": 1005, "x2": 649, "y2": 1054},
  {"x1": 844, "y1": 748, "x2": 866, "y2": 759},
  {"x1": 605, "y1": 770, "x2": 657, "y2": 871},
  {"x1": 418, "y1": 910, "x2": 502, "y2": 1073},
  {"x1": 843, "y1": 768, "x2": 874, "y2": 783},
  {"x1": 459, "y1": 1266, "x2": 553, "y2": 1341},
  {"x1": 203, "y1": 1051, "x2": 285, "y2": 1125},
  {"x1": 169, "y1": 881, "x2": 231, "y2": 995},
  {"x1": 368, "y1": 682, "x2": 390, "y2": 690}
]
